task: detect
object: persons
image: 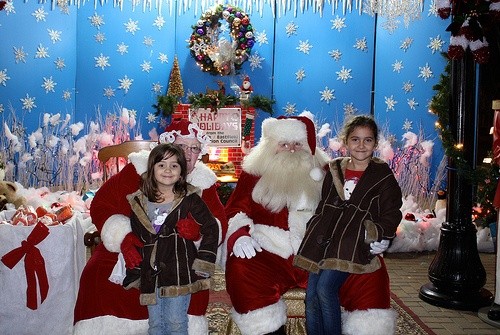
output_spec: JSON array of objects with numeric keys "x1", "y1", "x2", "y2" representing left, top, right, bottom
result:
[
  {"x1": 226, "y1": 116, "x2": 399, "y2": 335},
  {"x1": 293, "y1": 116, "x2": 404, "y2": 335},
  {"x1": 122, "y1": 143, "x2": 219, "y2": 335},
  {"x1": 74, "y1": 119, "x2": 228, "y2": 335}
]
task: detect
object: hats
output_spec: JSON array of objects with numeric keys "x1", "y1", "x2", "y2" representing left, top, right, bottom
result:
[
  {"x1": 261, "y1": 116, "x2": 322, "y2": 181},
  {"x1": 150, "y1": 118, "x2": 210, "y2": 159}
]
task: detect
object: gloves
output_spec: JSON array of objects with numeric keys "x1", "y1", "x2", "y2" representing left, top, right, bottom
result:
[
  {"x1": 234, "y1": 235, "x2": 262, "y2": 260},
  {"x1": 121, "y1": 232, "x2": 145, "y2": 270},
  {"x1": 369, "y1": 239, "x2": 390, "y2": 255},
  {"x1": 176, "y1": 213, "x2": 201, "y2": 242}
]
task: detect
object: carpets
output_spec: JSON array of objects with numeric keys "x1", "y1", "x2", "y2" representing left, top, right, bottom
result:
[{"x1": 207, "y1": 266, "x2": 437, "y2": 335}]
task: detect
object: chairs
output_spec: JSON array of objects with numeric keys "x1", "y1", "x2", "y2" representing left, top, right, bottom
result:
[{"x1": 83, "y1": 139, "x2": 157, "y2": 264}]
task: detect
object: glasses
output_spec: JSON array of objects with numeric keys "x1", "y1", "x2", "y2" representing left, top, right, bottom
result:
[
  {"x1": 277, "y1": 141, "x2": 304, "y2": 149},
  {"x1": 173, "y1": 144, "x2": 201, "y2": 154}
]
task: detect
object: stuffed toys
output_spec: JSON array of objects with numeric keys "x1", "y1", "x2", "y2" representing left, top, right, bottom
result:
[
  {"x1": 388, "y1": 193, "x2": 494, "y2": 253},
  {"x1": 0, "y1": 181, "x2": 95, "y2": 228},
  {"x1": 241, "y1": 75, "x2": 254, "y2": 95}
]
